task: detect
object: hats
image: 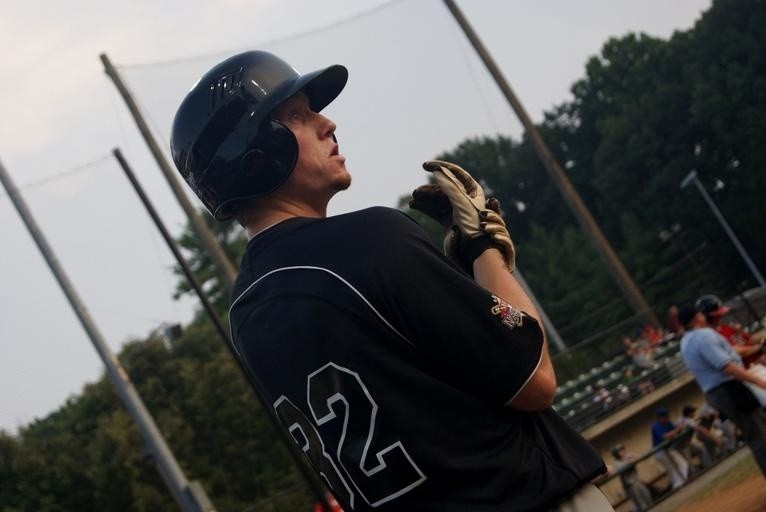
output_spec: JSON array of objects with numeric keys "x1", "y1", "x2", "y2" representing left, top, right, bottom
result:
[{"x1": 678, "y1": 294, "x2": 728, "y2": 326}]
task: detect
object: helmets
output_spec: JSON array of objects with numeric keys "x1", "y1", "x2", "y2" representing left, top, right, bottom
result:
[{"x1": 170, "y1": 50, "x2": 348, "y2": 221}]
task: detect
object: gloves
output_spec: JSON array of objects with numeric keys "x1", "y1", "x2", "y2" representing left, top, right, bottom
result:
[{"x1": 408, "y1": 159, "x2": 516, "y2": 280}]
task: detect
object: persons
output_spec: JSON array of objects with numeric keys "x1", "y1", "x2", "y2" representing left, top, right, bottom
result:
[
  {"x1": 313, "y1": 482, "x2": 344, "y2": 511},
  {"x1": 596, "y1": 293, "x2": 766, "y2": 508},
  {"x1": 169, "y1": 48, "x2": 614, "y2": 511}
]
